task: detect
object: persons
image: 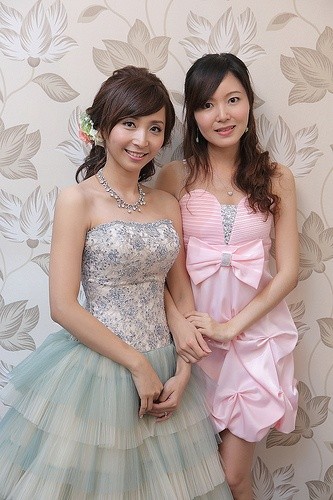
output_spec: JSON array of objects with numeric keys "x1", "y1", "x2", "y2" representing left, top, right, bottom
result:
[
  {"x1": 153, "y1": 51, "x2": 301, "y2": 500},
  {"x1": 0, "y1": 65, "x2": 235, "y2": 500}
]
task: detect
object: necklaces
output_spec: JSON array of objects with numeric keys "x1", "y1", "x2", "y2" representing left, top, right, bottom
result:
[
  {"x1": 211, "y1": 166, "x2": 236, "y2": 196},
  {"x1": 96, "y1": 167, "x2": 147, "y2": 215}
]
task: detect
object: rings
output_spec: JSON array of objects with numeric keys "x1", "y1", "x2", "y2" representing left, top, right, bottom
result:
[{"x1": 164, "y1": 412, "x2": 168, "y2": 418}]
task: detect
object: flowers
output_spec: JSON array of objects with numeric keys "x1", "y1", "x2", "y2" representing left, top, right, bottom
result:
[{"x1": 77, "y1": 109, "x2": 106, "y2": 149}]
